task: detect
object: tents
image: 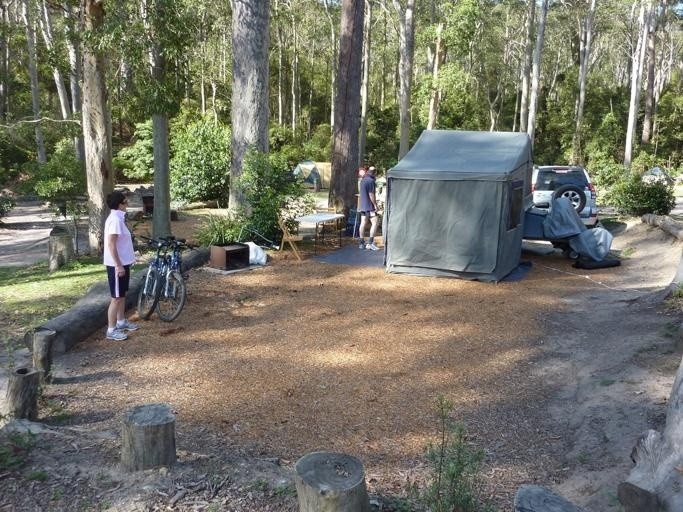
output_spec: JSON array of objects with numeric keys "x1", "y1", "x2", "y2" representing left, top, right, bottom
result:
[
  {"x1": 291, "y1": 160, "x2": 333, "y2": 191},
  {"x1": 377, "y1": 127, "x2": 536, "y2": 286}
]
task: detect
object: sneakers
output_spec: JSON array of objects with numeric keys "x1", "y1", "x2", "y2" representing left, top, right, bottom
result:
[
  {"x1": 359, "y1": 242, "x2": 380, "y2": 251},
  {"x1": 116, "y1": 319, "x2": 138, "y2": 332},
  {"x1": 106, "y1": 326, "x2": 128, "y2": 341}
]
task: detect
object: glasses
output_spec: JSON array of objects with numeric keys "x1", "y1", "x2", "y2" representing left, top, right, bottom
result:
[{"x1": 121, "y1": 201, "x2": 128, "y2": 205}]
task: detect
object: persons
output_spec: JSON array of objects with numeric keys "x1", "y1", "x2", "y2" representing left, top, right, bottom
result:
[
  {"x1": 102, "y1": 191, "x2": 139, "y2": 342},
  {"x1": 356, "y1": 166, "x2": 382, "y2": 252}
]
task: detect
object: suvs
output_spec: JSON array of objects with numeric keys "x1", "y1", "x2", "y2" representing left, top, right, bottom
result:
[{"x1": 529, "y1": 162, "x2": 599, "y2": 229}]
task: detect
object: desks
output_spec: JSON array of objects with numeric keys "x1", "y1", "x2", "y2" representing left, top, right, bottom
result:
[{"x1": 295, "y1": 211, "x2": 346, "y2": 256}]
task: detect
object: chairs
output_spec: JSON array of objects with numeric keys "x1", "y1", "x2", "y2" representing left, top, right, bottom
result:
[
  {"x1": 277, "y1": 216, "x2": 304, "y2": 262},
  {"x1": 312, "y1": 205, "x2": 339, "y2": 248}
]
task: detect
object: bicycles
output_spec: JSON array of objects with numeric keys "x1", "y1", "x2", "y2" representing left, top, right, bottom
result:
[
  {"x1": 155, "y1": 234, "x2": 199, "y2": 322},
  {"x1": 138, "y1": 232, "x2": 171, "y2": 323}
]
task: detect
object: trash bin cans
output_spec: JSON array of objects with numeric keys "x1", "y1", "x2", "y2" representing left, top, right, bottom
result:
[
  {"x1": 142, "y1": 196, "x2": 154, "y2": 219},
  {"x1": 314, "y1": 179, "x2": 320, "y2": 193}
]
